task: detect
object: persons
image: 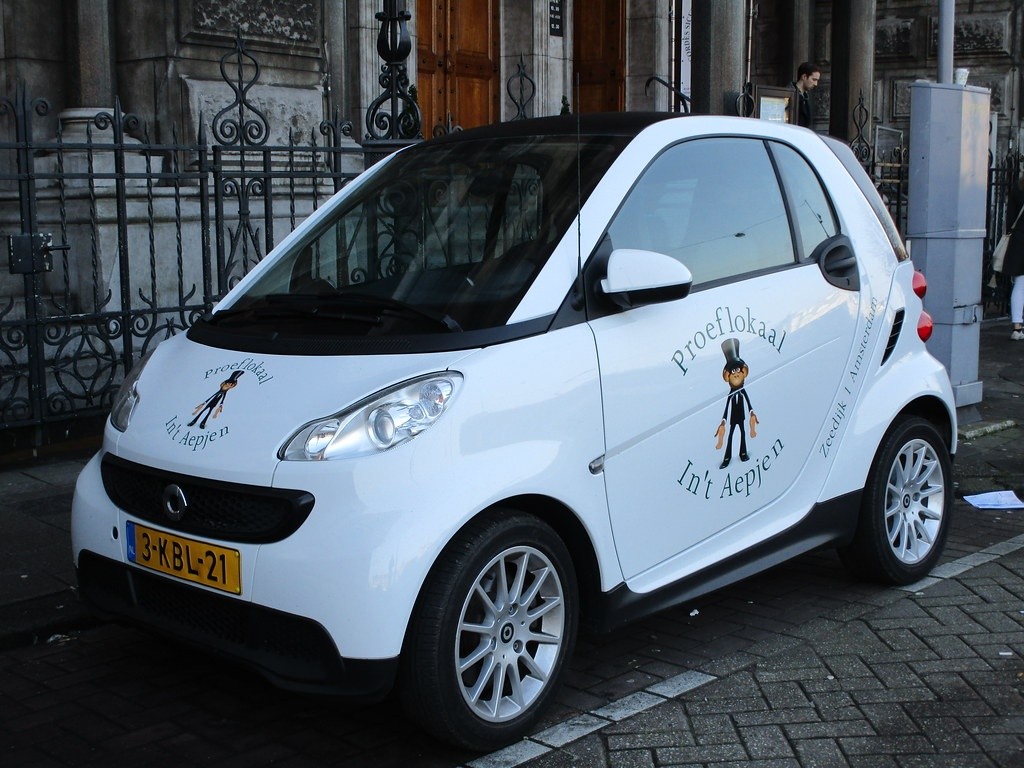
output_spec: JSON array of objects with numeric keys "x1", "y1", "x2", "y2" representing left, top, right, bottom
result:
[
  {"x1": 1009, "y1": 177, "x2": 1024, "y2": 340},
  {"x1": 786, "y1": 62, "x2": 820, "y2": 131}
]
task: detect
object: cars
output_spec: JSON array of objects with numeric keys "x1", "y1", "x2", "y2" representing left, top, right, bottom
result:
[{"x1": 66, "y1": 108, "x2": 960, "y2": 753}]
